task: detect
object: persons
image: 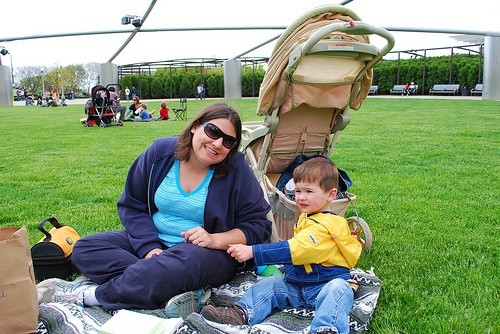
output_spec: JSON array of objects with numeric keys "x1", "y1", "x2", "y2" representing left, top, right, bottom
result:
[
  {"x1": 200, "y1": 157, "x2": 363, "y2": 334},
  {"x1": 101, "y1": 86, "x2": 126, "y2": 126},
  {"x1": 402, "y1": 81, "x2": 416, "y2": 96},
  {"x1": 135, "y1": 103, "x2": 149, "y2": 121},
  {"x1": 24, "y1": 88, "x2": 67, "y2": 107},
  {"x1": 124, "y1": 86, "x2": 137, "y2": 100},
  {"x1": 124, "y1": 96, "x2": 144, "y2": 119},
  {"x1": 196, "y1": 84, "x2": 205, "y2": 100},
  {"x1": 159, "y1": 103, "x2": 169, "y2": 120},
  {"x1": 16, "y1": 88, "x2": 27, "y2": 101},
  {"x1": 36, "y1": 102, "x2": 272, "y2": 318}
]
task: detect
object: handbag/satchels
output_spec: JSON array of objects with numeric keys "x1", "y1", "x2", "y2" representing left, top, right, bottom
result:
[
  {"x1": 30, "y1": 217, "x2": 82, "y2": 283},
  {"x1": 0, "y1": 225, "x2": 39, "y2": 334}
]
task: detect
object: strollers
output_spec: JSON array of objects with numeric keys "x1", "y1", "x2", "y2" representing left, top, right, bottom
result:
[
  {"x1": 84, "y1": 83, "x2": 122, "y2": 129},
  {"x1": 238, "y1": 3, "x2": 395, "y2": 254}
]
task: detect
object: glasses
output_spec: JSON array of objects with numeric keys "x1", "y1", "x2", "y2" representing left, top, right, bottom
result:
[
  {"x1": 199, "y1": 119, "x2": 237, "y2": 149},
  {"x1": 134, "y1": 99, "x2": 136, "y2": 101}
]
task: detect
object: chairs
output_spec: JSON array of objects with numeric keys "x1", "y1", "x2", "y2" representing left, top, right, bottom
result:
[{"x1": 171, "y1": 97, "x2": 187, "y2": 121}]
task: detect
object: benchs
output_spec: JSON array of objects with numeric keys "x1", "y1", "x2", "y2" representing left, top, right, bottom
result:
[
  {"x1": 369, "y1": 86, "x2": 379, "y2": 96},
  {"x1": 429, "y1": 84, "x2": 459, "y2": 96},
  {"x1": 470, "y1": 84, "x2": 484, "y2": 97},
  {"x1": 390, "y1": 84, "x2": 419, "y2": 96}
]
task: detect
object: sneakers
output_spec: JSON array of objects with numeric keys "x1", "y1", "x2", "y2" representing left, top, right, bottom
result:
[
  {"x1": 164, "y1": 291, "x2": 202, "y2": 318},
  {"x1": 202, "y1": 305, "x2": 247, "y2": 325},
  {"x1": 317, "y1": 329, "x2": 335, "y2": 334},
  {"x1": 36, "y1": 276, "x2": 98, "y2": 306}
]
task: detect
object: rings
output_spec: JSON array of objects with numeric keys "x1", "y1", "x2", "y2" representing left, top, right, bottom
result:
[{"x1": 196, "y1": 237, "x2": 200, "y2": 243}]
